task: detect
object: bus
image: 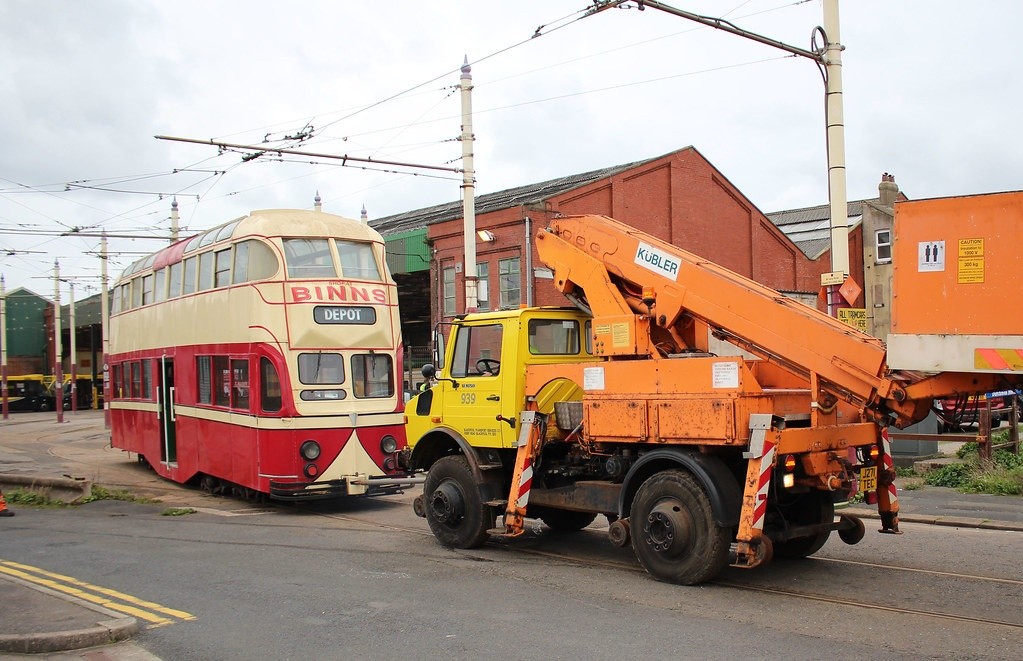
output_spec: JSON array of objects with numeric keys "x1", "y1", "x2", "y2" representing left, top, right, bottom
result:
[{"x1": 102, "y1": 206, "x2": 417, "y2": 509}]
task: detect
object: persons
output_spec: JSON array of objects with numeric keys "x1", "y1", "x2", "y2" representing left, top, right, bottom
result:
[
  {"x1": 482, "y1": 344, "x2": 538, "y2": 377},
  {"x1": 420, "y1": 379, "x2": 429, "y2": 391}
]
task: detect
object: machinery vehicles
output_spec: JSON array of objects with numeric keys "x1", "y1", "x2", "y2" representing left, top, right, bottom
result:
[
  {"x1": 396, "y1": 189, "x2": 1023, "y2": 587},
  {"x1": 0, "y1": 373, "x2": 104, "y2": 413}
]
